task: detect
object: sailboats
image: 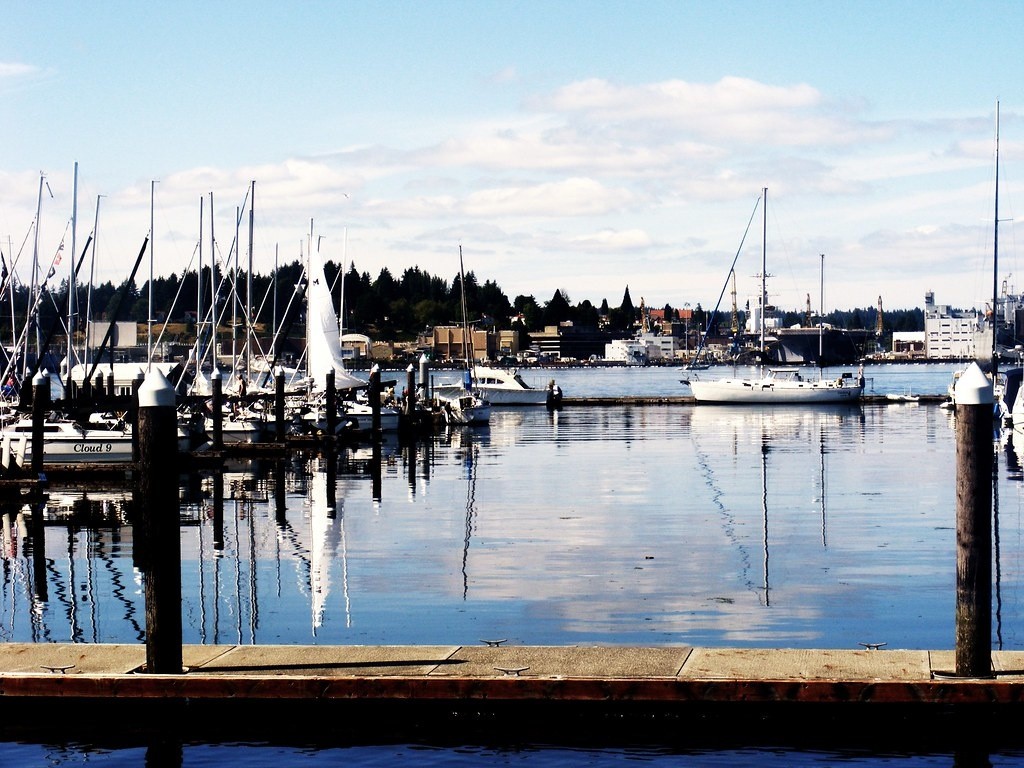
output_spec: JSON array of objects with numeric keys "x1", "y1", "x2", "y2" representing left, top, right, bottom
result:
[
  {"x1": 445, "y1": 245, "x2": 491, "y2": 426},
  {"x1": 0, "y1": 163, "x2": 452, "y2": 478},
  {"x1": 682, "y1": 187, "x2": 870, "y2": 406}
]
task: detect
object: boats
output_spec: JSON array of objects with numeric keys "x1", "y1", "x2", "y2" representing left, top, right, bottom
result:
[
  {"x1": 431, "y1": 366, "x2": 552, "y2": 406},
  {"x1": 683, "y1": 361, "x2": 711, "y2": 370},
  {"x1": 886, "y1": 387, "x2": 919, "y2": 402}
]
